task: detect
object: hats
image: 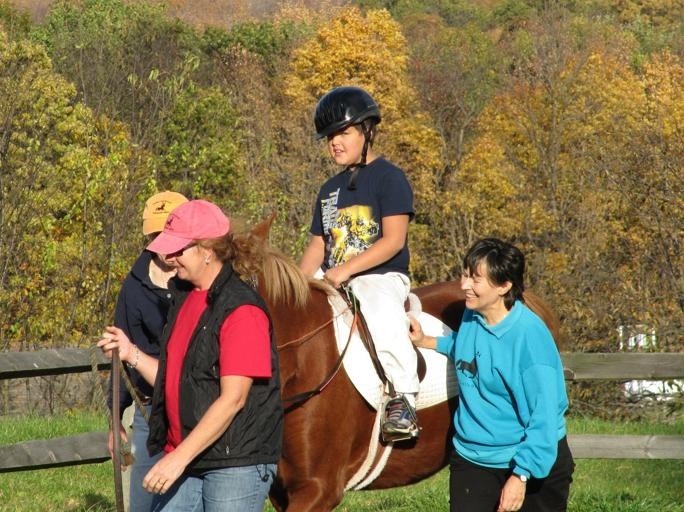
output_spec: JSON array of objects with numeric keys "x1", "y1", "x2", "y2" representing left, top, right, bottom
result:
[
  {"x1": 145, "y1": 199, "x2": 231, "y2": 256},
  {"x1": 142, "y1": 190, "x2": 191, "y2": 236}
]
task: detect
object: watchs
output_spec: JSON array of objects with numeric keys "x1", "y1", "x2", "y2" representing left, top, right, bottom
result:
[{"x1": 512, "y1": 472, "x2": 528, "y2": 481}]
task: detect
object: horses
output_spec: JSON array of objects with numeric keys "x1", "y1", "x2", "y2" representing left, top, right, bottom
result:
[{"x1": 238, "y1": 207, "x2": 566, "y2": 512}]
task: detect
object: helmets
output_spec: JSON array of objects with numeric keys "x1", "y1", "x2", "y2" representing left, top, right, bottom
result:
[{"x1": 313, "y1": 87, "x2": 381, "y2": 142}]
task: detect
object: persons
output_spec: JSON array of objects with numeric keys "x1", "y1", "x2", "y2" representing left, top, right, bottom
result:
[
  {"x1": 94, "y1": 197, "x2": 283, "y2": 510},
  {"x1": 407, "y1": 234, "x2": 575, "y2": 510},
  {"x1": 297, "y1": 85, "x2": 422, "y2": 446},
  {"x1": 105, "y1": 188, "x2": 188, "y2": 510}
]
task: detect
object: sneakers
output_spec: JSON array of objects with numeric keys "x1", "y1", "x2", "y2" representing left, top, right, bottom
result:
[{"x1": 380, "y1": 396, "x2": 421, "y2": 436}]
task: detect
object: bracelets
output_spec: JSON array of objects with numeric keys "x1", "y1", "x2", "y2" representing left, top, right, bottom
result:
[{"x1": 125, "y1": 342, "x2": 140, "y2": 369}]
